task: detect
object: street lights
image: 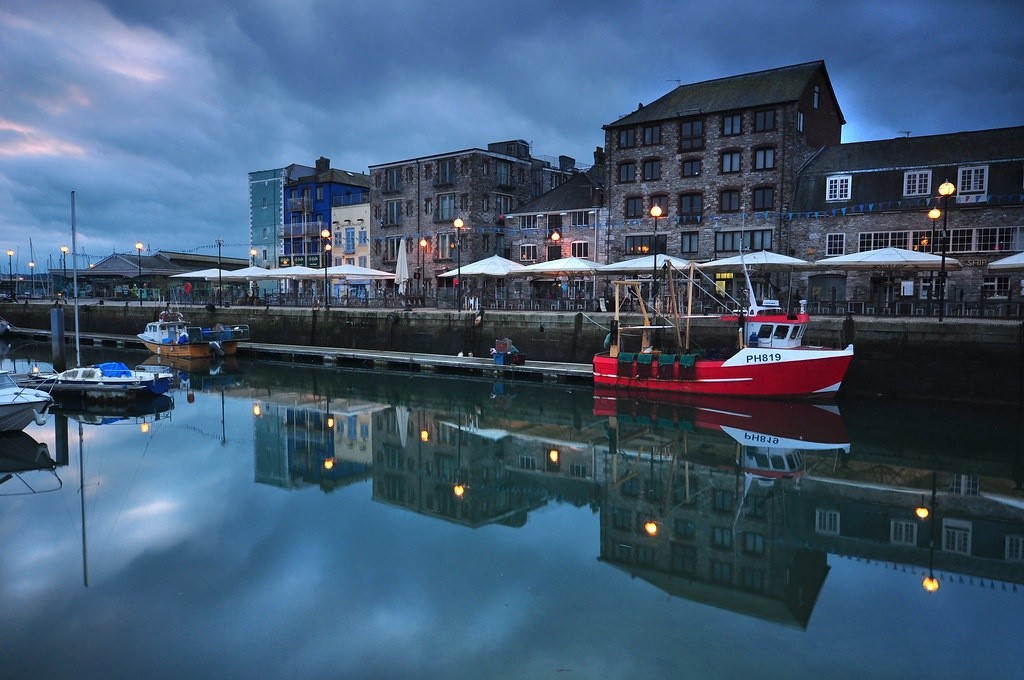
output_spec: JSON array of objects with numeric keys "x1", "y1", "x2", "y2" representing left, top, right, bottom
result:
[
  {"x1": 454, "y1": 217, "x2": 464, "y2": 311},
  {"x1": 216, "y1": 236, "x2": 224, "y2": 307},
  {"x1": 135, "y1": 242, "x2": 143, "y2": 305},
  {"x1": 29, "y1": 262, "x2": 35, "y2": 294},
  {"x1": 938, "y1": 181, "x2": 955, "y2": 320},
  {"x1": 61, "y1": 245, "x2": 68, "y2": 304},
  {"x1": 321, "y1": 229, "x2": 331, "y2": 307},
  {"x1": 650, "y1": 204, "x2": 663, "y2": 317},
  {"x1": 551, "y1": 230, "x2": 561, "y2": 303},
  {"x1": 251, "y1": 249, "x2": 257, "y2": 299},
  {"x1": 926, "y1": 208, "x2": 942, "y2": 317},
  {"x1": 420, "y1": 239, "x2": 427, "y2": 308},
  {"x1": 8, "y1": 249, "x2": 13, "y2": 298}
]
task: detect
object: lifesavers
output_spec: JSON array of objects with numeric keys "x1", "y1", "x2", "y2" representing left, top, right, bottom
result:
[
  {"x1": 178, "y1": 313, "x2": 183, "y2": 321},
  {"x1": 387, "y1": 314, "x2": 397, "y2": 324},
  {"x1": 159, "y1": 311, "x2": 168, "y2": 321}
]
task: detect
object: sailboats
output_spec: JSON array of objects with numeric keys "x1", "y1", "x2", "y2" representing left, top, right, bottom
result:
[
  {"x1": 15, "y1": 190, "x2": 173, "y2": 398},
  {"x1": 40, "y1": 395, "x2": 176, "y2": 586}
]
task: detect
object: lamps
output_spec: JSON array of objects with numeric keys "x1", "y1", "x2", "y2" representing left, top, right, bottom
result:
[
  {"x1": 537, "y1": 215, "x2": 544, "y2": 217},
  {"x1": 345, "y1": 219, "x2": 351, "y2": 222},
  {"x1": 588, "y1": 211, "x2": 595, "y2": 215},
  {"x1": 357, "y1": 218, "x2": 363, "y2": 221},
  {"x1": 506, "y1": 216, "x2": 514, "y2": 219},
  {"x1": 333, "y1": 221, "x2": 339, "y2": 224},
  {"x1": 559, "y1": 213, "x2": 568, "y2": 216}
]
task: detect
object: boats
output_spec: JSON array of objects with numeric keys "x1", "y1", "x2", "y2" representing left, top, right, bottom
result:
[
  {"x1": 592, "y1": 235, "x2": 854, "y2": 395},
  {"x1": 135, "y1": 353, "x2": 245, "y2": 403},
  {"x1": 137, "y1": 302, "x2": 251, "y2": 358},
  {"x1": 593, "y1": 387, "x2": 854, "y2": 530},
  {"x1": 0, "y1": 359, "x2": 60, "y2": 435},
  {"x1": 0, "y1": 432, "x2": 62, "y2": 497}
]
